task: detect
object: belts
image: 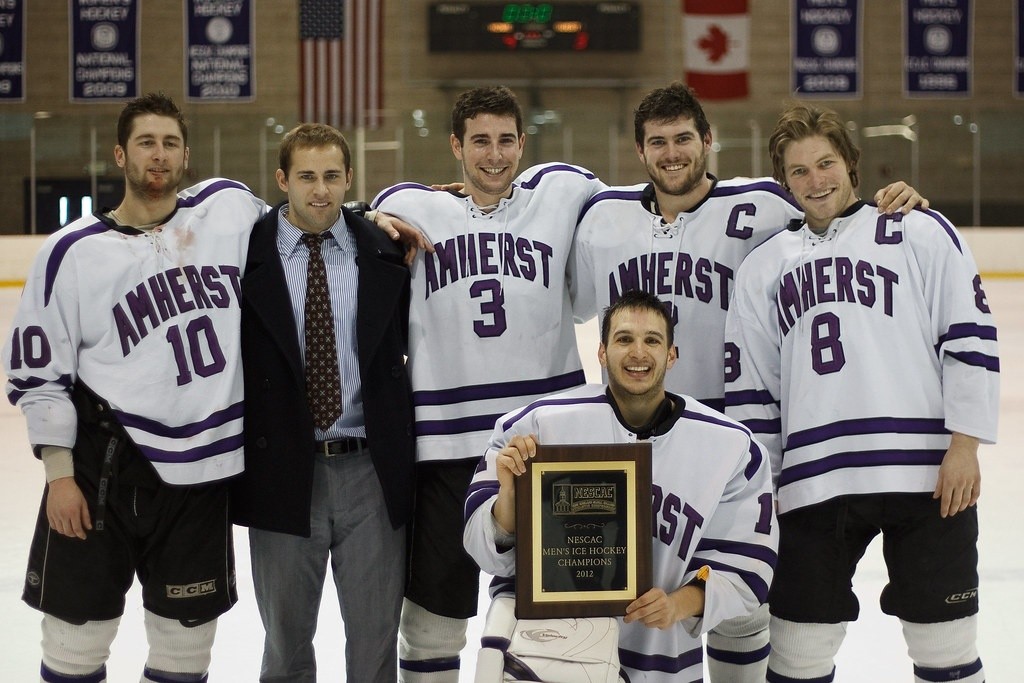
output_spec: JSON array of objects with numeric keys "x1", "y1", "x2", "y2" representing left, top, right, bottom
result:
[{"x1": 314, "y1": 437, "x2": 369, "y2": 456}]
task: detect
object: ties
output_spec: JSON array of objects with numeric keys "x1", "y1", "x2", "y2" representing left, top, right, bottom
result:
[{"x1": 302, "y1": 232, "x2": 344, "y2": 432}]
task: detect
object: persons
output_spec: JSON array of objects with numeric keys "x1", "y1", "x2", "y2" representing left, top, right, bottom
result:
[{"x1": 3, "y1": 81, "x2": 999, "y2": 683}]
